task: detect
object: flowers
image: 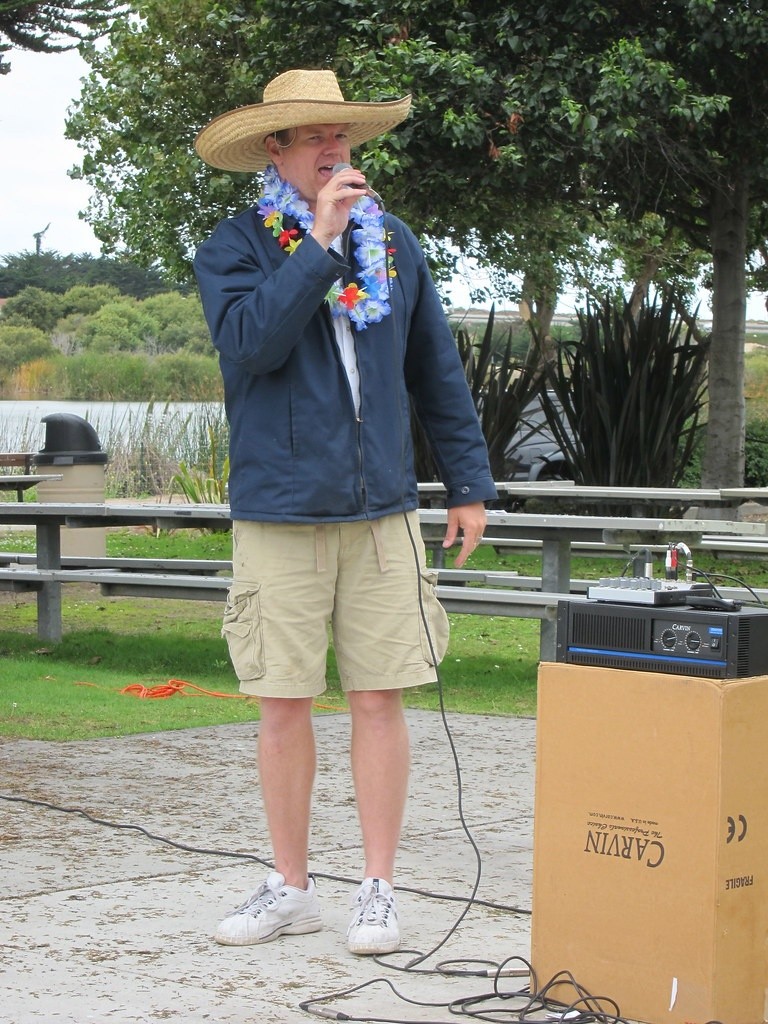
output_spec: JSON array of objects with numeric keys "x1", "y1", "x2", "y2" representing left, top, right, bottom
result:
[{"x1": 254, "y1": 162, "x2": 398, "y2": 332}]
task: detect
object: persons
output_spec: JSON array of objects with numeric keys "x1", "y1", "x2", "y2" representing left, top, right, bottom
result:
[{"x1": 192, "y1": 69, "x2": 499, "y2": 955}]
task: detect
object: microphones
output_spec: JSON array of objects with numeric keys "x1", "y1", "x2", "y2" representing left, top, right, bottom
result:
[{"x1": 332, "y1": 162, "x2": 382, "y2": 204}]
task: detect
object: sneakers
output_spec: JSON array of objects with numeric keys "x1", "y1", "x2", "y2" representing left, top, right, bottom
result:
[
  {"x1": 215, "y1": 870, "x2": 323, "y2": 946},
  {"x1": 347, "y1": 878, "x2": 401, "y2": 954}
]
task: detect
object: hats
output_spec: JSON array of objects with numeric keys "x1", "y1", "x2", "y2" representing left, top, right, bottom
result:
[{"x1": 192, "y1": 69, "x2": 411, "y2": 172}]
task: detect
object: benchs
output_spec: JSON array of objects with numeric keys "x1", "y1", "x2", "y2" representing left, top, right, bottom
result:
[{"x1": 0, "y1": 547, "x2": 768, "y2": 675}]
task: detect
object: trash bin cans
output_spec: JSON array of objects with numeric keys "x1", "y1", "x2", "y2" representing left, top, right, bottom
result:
[{"x1": 34, "y1": 413, "x2": 108, "y2": 568}]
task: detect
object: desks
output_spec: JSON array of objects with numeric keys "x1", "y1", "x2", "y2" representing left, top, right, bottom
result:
[{"x1": 1, "y1": 477, "x2": 768, "y2": 668}]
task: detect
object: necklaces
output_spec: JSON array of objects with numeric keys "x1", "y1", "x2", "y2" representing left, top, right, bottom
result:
[{"x1": 253, "y1": 158, "x2": 395, "y2": 333}]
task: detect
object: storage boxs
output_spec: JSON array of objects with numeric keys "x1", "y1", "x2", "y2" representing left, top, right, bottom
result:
[{"x1": 527, "y1": 660, "x2": 768, "y2": 1023}]
